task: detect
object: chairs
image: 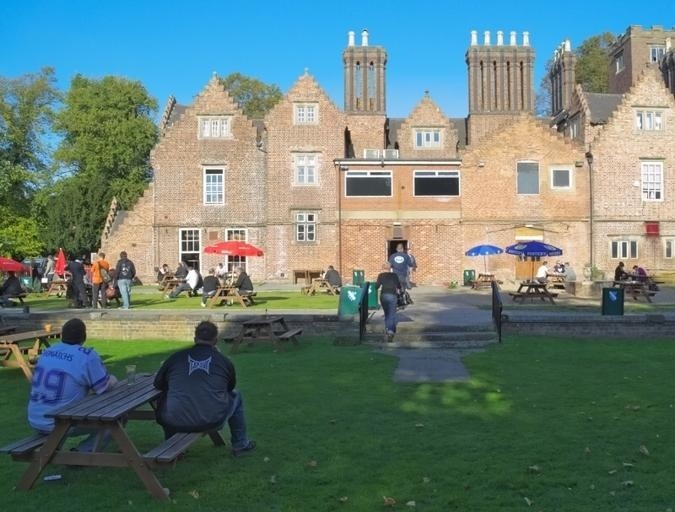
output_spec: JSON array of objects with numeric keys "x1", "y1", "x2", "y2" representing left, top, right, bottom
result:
[
  {"x1": 1, "y1": 271, "x2": 133, "y2": 308},
  {"x1": 154, "y1": 271, "x2": 259, "y2": 308},
  {"x1": 291, "y1": 270, "x2": 337, "y2": 298}
]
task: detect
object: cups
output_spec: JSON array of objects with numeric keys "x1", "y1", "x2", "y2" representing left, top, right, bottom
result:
[
  {"x1": 45, "y1": 324, "x2": 52, "y2": 333},
  {"x1": 125, "y1": 365, "x2": 136, "y2": 386}
]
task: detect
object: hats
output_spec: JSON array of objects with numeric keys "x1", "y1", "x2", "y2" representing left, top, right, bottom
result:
[
  {"x1": 195, "y1": 320, "x2": 218, "y2": 340},
  {"x1": 62, "y1": 318, "x2": 85, "y2": 344}
]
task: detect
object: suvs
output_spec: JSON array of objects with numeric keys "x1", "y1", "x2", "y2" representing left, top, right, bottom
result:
[{"x1": 2, "y1": 255, "x2": 51, "y2": 286}]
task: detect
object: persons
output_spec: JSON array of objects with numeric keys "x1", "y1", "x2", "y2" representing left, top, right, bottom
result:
[
  {"x1": 614, "y1": 261, "x2": 627, "y2": 281},
  {"x1": 0, "y1": 271, "x2": 22, "y2": 307},
  {"x1": 154, "y1": 321, "x2": 258, "y2": 458},
  {"x1": 388, "y1": 243, "x2": 412, "y2": 305},
  {"x1": 154, "y1": 258, "x2": 254, "y2": 307},
  {"x1": 554, "y1": 260, "x2": 565, "y2": 273},
  {"x1": 324, "y1": 266, "x2": 340, "y2": 295},
  {"x1": 535, "y1": 261, "x2": 556, "y2": 289},
  {"x1": 27, "y1": 319, "x2": 129, "y2": 467},
  {"x1": 560, "y1": 262, "x2": 577, "y2": 282},
  {"x1": 406, "y1": 248, "x2": 416, "y2": 288},
  {"x1": 44, "y1": 255, "x2": 136, "y2": 311},
  {"x1": 375, "y1": 260, "x2": 403, "y2": 343},
  {"x1": 634, "y1": 265, "x2": 648, "y2": 282}
]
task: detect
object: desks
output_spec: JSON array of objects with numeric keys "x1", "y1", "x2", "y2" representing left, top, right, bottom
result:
[
  {"x1": 0, "y1": 371, "x2": 225, "y2": 498},
  {"x1": 0, "y1": 327, "x2": 63, "y2": 384},
  {"x1": 226, "y1": 315, "x2": 298, "y2": 354}
]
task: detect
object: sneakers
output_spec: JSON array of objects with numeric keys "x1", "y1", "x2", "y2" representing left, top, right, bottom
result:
[{"x1": 233, "y1": 440, "x2": 255, "y2": 453}]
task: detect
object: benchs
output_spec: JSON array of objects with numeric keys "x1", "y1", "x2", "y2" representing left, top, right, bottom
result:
[
  {"x1": 470, "y1": 271, "x2": 664, "y2": 304},
  {"x1": 276, "y1": 327, "x2": 303, "y2": 340},
  {"x1": 142, "y1": 427, "x2": 206, "y2": 470},
  {"x1": 1, "y1": 426, "x2": 48, "y2": 463},
  {"x1": 222, "y1": 327, "x2": 258, "y2": 341}
]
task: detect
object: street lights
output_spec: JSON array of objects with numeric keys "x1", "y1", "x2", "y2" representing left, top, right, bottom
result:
[{"x1": 583, "y1": 142, "x2": 597, "y2": 277}]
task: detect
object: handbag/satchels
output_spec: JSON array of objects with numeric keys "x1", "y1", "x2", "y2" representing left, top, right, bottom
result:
[
  {"x1": 398, "y1": 288, "x2": 412, "y2": 306},
  {"x1": 105, "y1": 288, "x2": 118, "y2": 298},
  {"x1": 98, "y1": 262, "x2": 112, "y2": 283}
]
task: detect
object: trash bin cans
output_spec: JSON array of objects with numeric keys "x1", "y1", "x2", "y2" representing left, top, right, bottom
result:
[
  {"x1": 603, "y1": 288, "x2": 623, "y2": 315},
  {"x1": 367, "y1": 282, "x2": 380, "y2": 310},
  {"x1": 353, "y1": 270, "x2": 364, "y2": 288},
  {"x1": 338, "y1": 287, "x2": 362, "y2": 314},
  {"x1": 464, "y1": 270, "x2": 475, "y2": 285}
]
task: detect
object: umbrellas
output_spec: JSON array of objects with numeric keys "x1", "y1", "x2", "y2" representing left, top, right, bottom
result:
[
  {"x1": 0, "y1": 256, "x2": 26, "y2": 272},
  {"x1": 55, "y1": 248, "x2": 68, "y2": 276},
  {"x1": 204, "y1": 240, "x2": 263, "y2": 280},
  {"x1": 506, "y1": 240, "x2": 563, "y2": 280},
  {"x1": 465, "y1": 244, "x2": 504, "y2": 273}
]
